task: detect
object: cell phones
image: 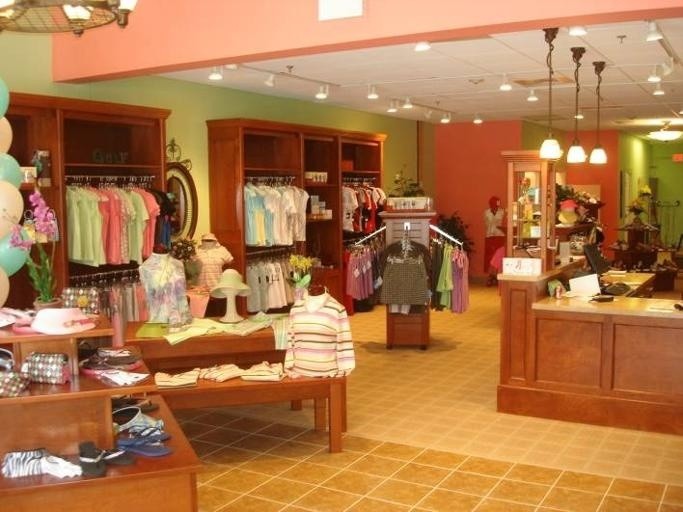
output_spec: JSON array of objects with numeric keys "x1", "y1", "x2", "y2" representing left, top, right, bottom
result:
[{"x1": 590, "y1": 296, "x2": 615, "y2": 303}]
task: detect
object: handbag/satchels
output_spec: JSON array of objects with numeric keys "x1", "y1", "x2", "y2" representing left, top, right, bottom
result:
[
  {"x1": 22, "y1": 351, "x2": 70, "y2": 385},
  {"x1": 0, "y1": 371, "x2": 32, "y2": 397}
]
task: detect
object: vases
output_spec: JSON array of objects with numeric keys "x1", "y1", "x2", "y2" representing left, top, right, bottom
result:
[
  {"x1": 32, "y1": 299, "x2": 62, "y2": 310},
  {"x1": 290, "y1": 300, "x2": 305, "y2": 314},
  {"x1": 570, "y1": 231, "x2": 588, "y2": 256}
]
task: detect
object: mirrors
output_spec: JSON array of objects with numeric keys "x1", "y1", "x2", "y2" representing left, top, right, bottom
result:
[{"x1": 512, "y1": 168, "x2": 543, "y2": 259}]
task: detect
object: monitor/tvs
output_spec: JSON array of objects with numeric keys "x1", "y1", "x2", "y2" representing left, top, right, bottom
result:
[{"x1": 583, "y1": 242, "x2": 610, "y2": 276}]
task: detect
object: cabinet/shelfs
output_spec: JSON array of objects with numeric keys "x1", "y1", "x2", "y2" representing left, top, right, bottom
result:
[
  {"x1": 555, "y1": 221, "x2": 592, "y2": 252},
  {"x1": 0, "y1": 317, "x2": 202, "y2": 511},
  {"x1": 609, "y1": 227, "x2": 678, "y2": 291},
  {"x1": 497, "y1": 255, "x2": 682, "y2": 435},
  {"x1": 355, "y1": 213, "x2": 463, "y2": 349},
  {"x1": 240, "y1": 128, "x2": 302, "y2": 315},
  {"x1": 341, "y1": 135, "x2": 383, "y2": 244},
  {"x1": 63, "y1": 109, "x2": 165, "y2": 307},
  {"x1": 305, "y1": 134, "x2": 339, "y2": 275},
  {"x1": 5, "y1": 103, "x2": 62, "y2": 310},
  {"x1": 125, "y1": 320, "x2": 348, "y2": 455}
]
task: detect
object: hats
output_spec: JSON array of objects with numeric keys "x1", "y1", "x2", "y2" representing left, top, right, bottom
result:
[
  {"x1": 203, "y1": 232, "x2": 216, "y2": 241},
  {"x1": 561, "y1": 200, "x2": 577, "y2": 210},
  {"x1": 31, "y1": 308, "x2": 95, "y2": 335},
  {"x1": 112, "y1": 407, "x2": 164, "y2": 433},
  {"x1": 211, "y1": 269, "x2": 252, "y2": 299}
]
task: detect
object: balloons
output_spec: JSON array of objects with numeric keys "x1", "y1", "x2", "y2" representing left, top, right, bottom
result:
[
  {"x1": 2, "y1": 151, "x2": 22, "y2": 186},
  {"x1": 1, "y1": 116, "x2": 12, "y2": 157},
  {"x1": 1, "y1": 268, "x2": 10, "y2": 305},
  {"x1": 1, "y1": 181, "x2": 23, "y2": 225},
  {"x1": 0, "y1": 79, "x2": 10, "y2": 120},
  {"x1": 0, "y1": 226, "x2": 29, "y2": 273}
]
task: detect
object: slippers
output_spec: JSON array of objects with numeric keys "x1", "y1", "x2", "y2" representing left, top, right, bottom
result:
[
  {"x1": 120, "y1": 445, "x2": 174, "y2": 455},
  {"x1": 117, "y1": 427, "x2": 169, "y2": 445},
  {"x1": 102, "y1": 449, "x2": 133, "y2": 465},
  {"x1": 80, "y1": 440, "x2": 107, "y2": 477},
  {"x1": 114, "y1": 398, "x2": 157, "y2": 412}
]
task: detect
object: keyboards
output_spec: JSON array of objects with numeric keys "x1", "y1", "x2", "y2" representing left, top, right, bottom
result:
[{"x1": 605, "y1": 281, "x2": 630, "y2": 296}]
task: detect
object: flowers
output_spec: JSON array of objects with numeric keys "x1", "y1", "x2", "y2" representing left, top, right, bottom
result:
[
  {"x1": 170, "y1": 235, "x2": 197, "y2": 265},
  {"x1": 12, "y1": 195, "x2": 61, "y2": 300},
  {"x1": 575, "y1": 206, "x2": 590, "y2": 224},
  {"x1": 286, "y1": 253, "x2": 316, "y2": 289}
]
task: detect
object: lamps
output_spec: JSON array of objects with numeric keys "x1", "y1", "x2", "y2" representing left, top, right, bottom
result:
[
  {"x1": 566, "y1": 47, "x2": 587, "y2": 162},
  {"x1": 424, "y1": 110, "x2": 434, "y2": 118},
  {"x1": 403, "y1": 98, "x2": 412, "y2": 108},
  {"x1": 387, "y1": 100, "x2": 397, "y2": 112},
  {"x1": 315, "y1": 84, "x2": 328, "y2": 99},
  {"x1": 440, "y1": 111, "x2": 451, "y2": 123},
  {"x1": 500, "y1": 74, "x2": 512, "y2": 91},
  {"x1": 653, "y1": 82, "x2": 666, "y2": 95},
  {"x1": 573, "y1": 111, "x2": 584, "y2": 118},
  {"x1": 368, "y1": 85, "x2": 378, "y2": 99},
  {"x1": 649, "y1": 65, "x2": 662, "y2": 82},
  {"x1": 646, "y1": 21, "x2": 663, "y2": 42},
  {"x1": 417, "y1": 43, "x2": 433, "y2": 52},
  {"x1": 568, "y1": 24, "x2": 588, "y2": 35},
  {"x1": 0, "y1": 1, "x2": 136, "y2": 35},
  {"x1": 209, "y1": 65, "x2": 223, "y2": 81},
  {"x1": 589, "y1": 60, "x2": 607, "y2": 165},
  {"x1": 527, "y1": 87, "x2": 539, "y2": 101},
  {"x1": 473, "y1": 115, "x2": 484, "y2": 123},
  {"x1": 646, "y1": 120, "x2": 682, "y2": 141},
  {"x1": 662, "y1": 62, "x2": 671, "y2": 76},
  {"x1": 265, "y1": 74, "x2": 274, "y2": 87},
  {"x1": 540, "y1": 27, "x2": 561, "y2": 158}
]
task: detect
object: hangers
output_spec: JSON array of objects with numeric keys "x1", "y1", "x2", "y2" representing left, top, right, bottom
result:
[
  {"x1": 73, "y1": 269, "x2": 140, "y2": 288},
  {"x1": 246, "y1": 174, "x2": 293, "y2": 188},
  {"x1": 355, "y1": 226, "x2": 383, "y2": 256},
  {"x1": 434, "y1": 228, "x2": 463, "y2": 250},
  {"x1": 344, "y1": 176, "x2": 378, "y2": 184},
  {"x1": 63, "y1": 175, "x2": 156, "y2": 190}
]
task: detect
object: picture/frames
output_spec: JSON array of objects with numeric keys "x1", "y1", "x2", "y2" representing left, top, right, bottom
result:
[{"x1": 620, "y1": 169, "x2": 632, "y2": 217}]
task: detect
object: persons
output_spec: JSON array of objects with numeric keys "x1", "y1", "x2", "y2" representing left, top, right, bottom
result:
[{"x1": 483, "y1": 196, "x2": 507, "y2": 287}]
